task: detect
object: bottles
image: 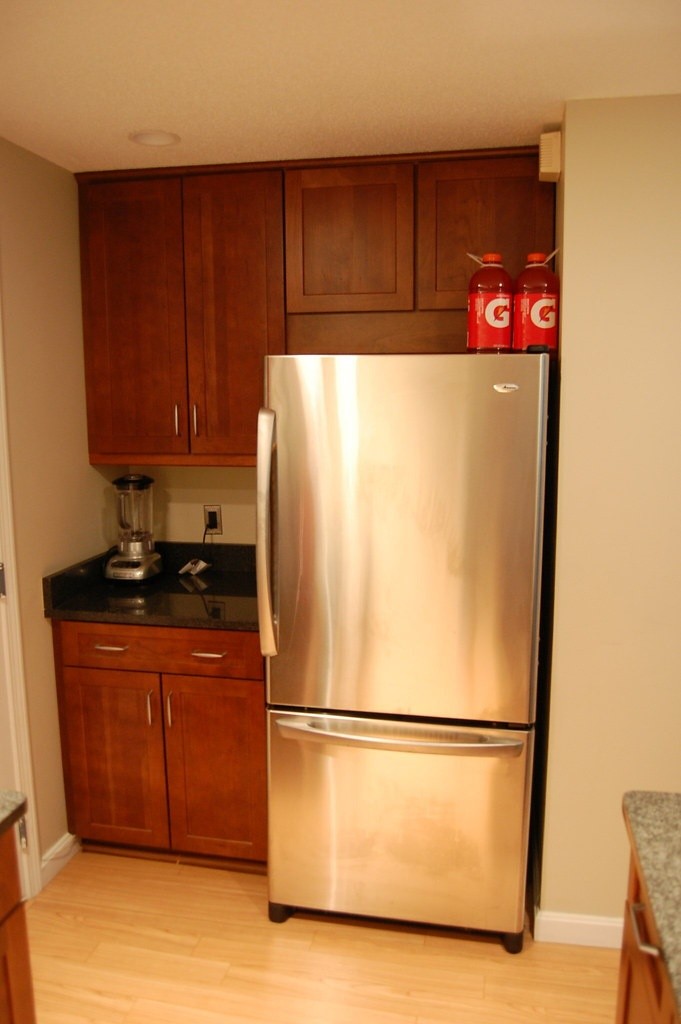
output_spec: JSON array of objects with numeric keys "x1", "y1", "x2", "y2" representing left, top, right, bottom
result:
[{"x1": 466, "y1": 252, "x2": 558, "y2": 353}]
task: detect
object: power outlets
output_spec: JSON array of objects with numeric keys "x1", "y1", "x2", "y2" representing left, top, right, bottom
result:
[{"x1": 203, "y1": 505, "x2": 223, "y2": 535}]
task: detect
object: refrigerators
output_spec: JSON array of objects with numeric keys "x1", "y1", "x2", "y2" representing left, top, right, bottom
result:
[{"x1": 256, "y1": 354, "x2": 552, "y2": 956}]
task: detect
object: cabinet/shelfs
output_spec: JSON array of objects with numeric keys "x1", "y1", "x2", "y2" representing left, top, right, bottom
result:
[
  {"x1": 616, "y1": 790, "x2": 681, "y2": 1024},
  {"x1": 43, "y1": 541, "x2": 270, "y2": 875},
  {"x1": 70, "y1": 146, "x2": 556, "y2": 466},
  {"x1": 0, "y1": 790, "x2": 42, "y2": 1024}
]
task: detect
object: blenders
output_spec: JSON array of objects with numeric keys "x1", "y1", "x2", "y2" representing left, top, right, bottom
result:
[{"x1": 107, "y1": 475, "x2": 165, "y2": 580}]
